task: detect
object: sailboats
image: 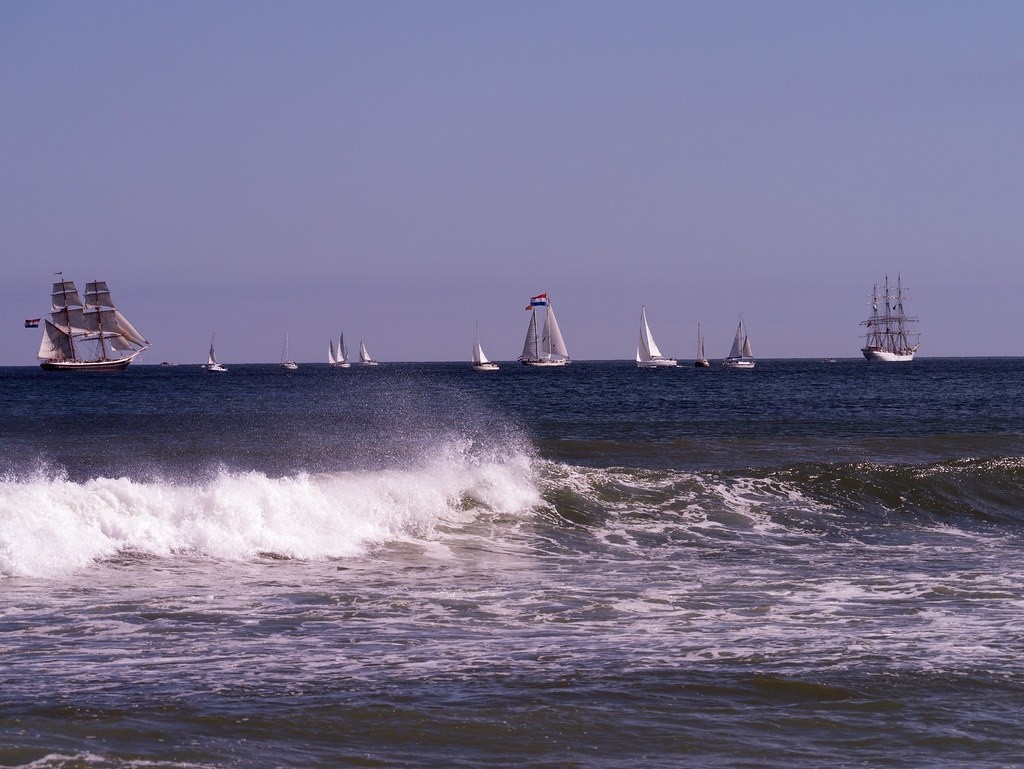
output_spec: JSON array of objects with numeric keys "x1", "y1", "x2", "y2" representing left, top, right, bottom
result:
[
  {"x1": 279, "y1": 332, "x2": 298, "y2": 370},
  {"x1": 334, "y1": 330, "x2": 351, "y2": 369},
  {"x1": 470, "y1": 320, "x2": 499, "y2": 372},
  {"x1": 858, "y1": 271, "x2": 923, "y2": 363},
  {"x1": 356, "y1": 340, "x2": 378, "y2": 366},
  {"x1": 635, "y1": 304, "x2": 677, "y2": 368},
  {"x1": 24, "y1": 271, "x2": 152, "y2": 372},
  {"x1": 514, "y1": 291, "x2": 572, "y2": 367},
  {"x1": 720, "y1": 313, "x2": 756, "y2": 369},
  {"x1": 694, "y1": 321, "x2": 710, "y2": 368},
  {"x1": 206, "y1": 330, "x2": 229, "y2": 372},
  {"x1": 327, "y1": 339, "x2": 337, "y2": 367}
]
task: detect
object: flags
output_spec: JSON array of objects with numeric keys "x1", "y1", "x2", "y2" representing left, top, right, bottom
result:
[
  {"x1": 525, "y1": 306, "x2": 533, "y2": 310},
  {"x1": 25, "y1": 319, "x2": 41, "y2": 328},
  {"x1": 531, "y1": 294, "x2": 547, "y2": 306}
]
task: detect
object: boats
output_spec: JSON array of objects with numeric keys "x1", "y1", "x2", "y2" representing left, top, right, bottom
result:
[
  {"x1": 818, "y1": 357, "x2": 837, "y2": 363},
  {"x1": 159, "y1": 359, "x2": 179, "y2": 369},
  {"x1": 197, "y1": 364, "x2": 205, "y2": 368}
]
